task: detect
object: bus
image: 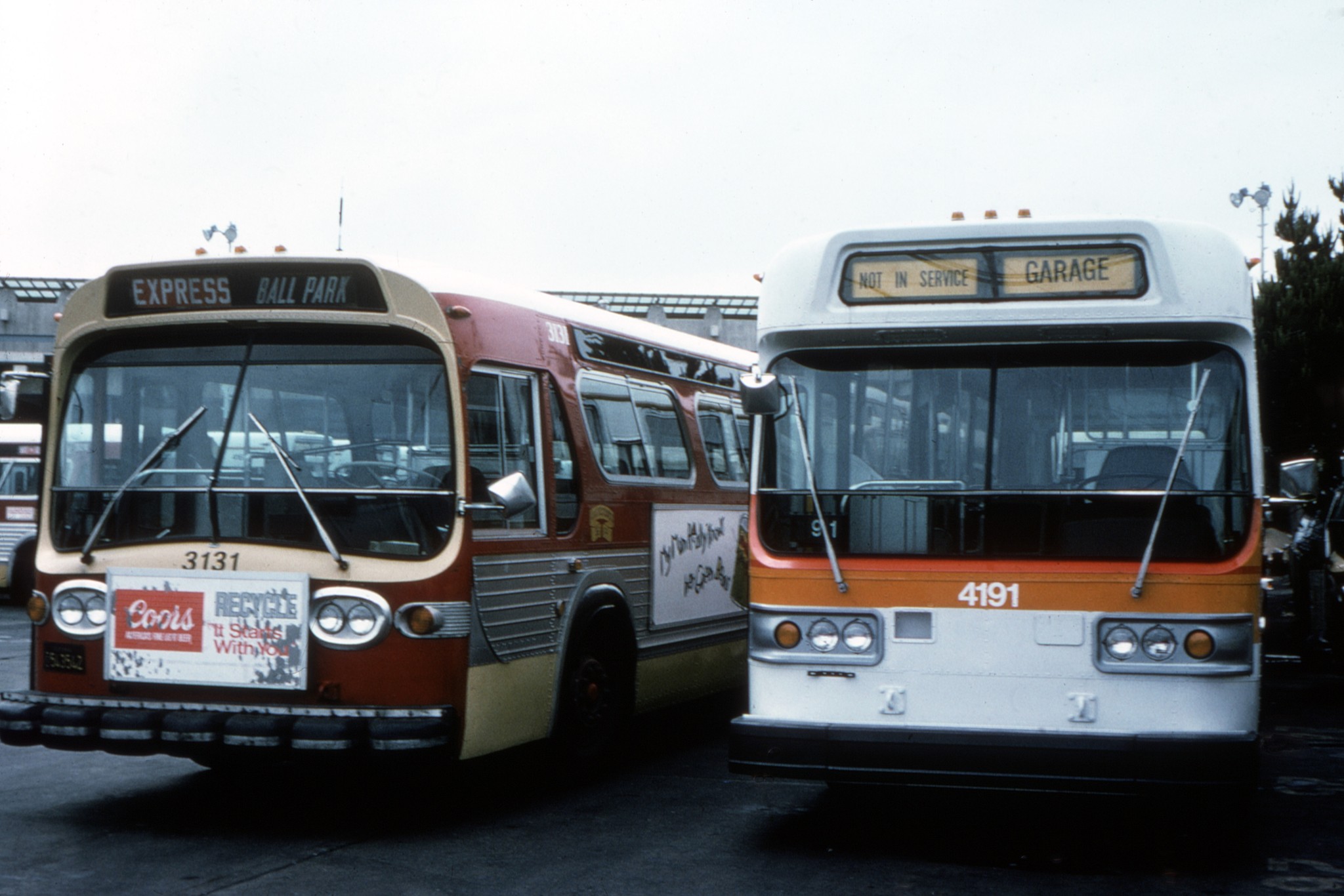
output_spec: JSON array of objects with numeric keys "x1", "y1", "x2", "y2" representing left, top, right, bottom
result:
[
  {"x1": 0, "y1": 255, "x2": 966, "y2": 805},
  {"x1": 716, "y1": 215, "x2": 1326, "y2": 830},
  {"x1": 0, "y1": 369, "x2": 430, "y2": 612}
]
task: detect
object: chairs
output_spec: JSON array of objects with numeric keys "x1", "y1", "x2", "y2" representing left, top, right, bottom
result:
[{"x1": 1094, "y1": 446, "x2": 1198, "y2": 506}]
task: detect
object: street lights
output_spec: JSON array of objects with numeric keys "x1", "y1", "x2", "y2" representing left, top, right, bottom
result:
[
  {"x1": 1229, "y1": 181, "x2": 1272, "y2": 283},
  {"x1": 202, "y1": 222, "x2": 237, "y2": 253}
]
